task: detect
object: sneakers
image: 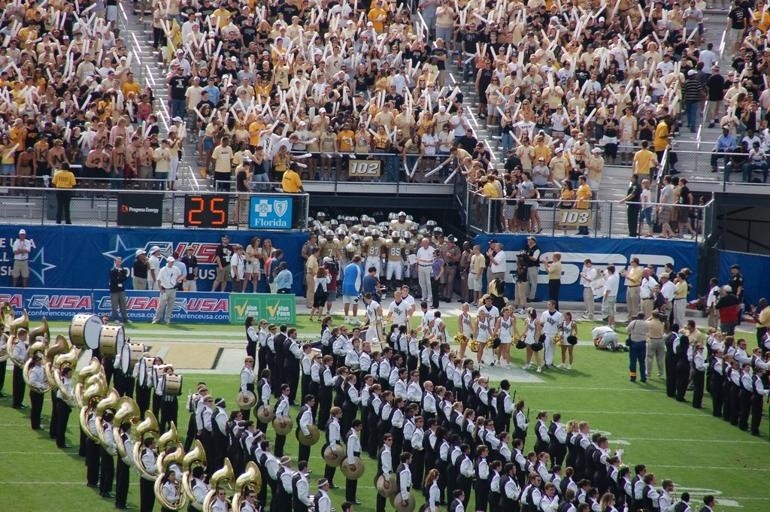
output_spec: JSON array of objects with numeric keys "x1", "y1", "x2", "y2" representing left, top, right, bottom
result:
[{"x1": 477, "y1": 360, "x2": 572, "y2": 372}]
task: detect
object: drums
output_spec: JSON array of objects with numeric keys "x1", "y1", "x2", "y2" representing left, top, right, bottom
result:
[
  {"x1": 121, "y1": 343, "x2": 182, "y2": 394},
  {"x1": 307, "y1": 495, "x2": 316, "y2": 512}
]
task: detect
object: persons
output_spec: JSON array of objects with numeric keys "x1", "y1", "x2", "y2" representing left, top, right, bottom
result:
[{"x1": 1, "y1": 1, "x2": 768, "y2": 509}]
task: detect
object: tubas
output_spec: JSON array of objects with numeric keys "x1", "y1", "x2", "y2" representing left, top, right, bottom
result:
[
  {"x1": 0, "y1": 302, "x2": 140, "y2": 467},
  {"x1": 133, "y1": 410, "x2": 262, "y2": 512}
]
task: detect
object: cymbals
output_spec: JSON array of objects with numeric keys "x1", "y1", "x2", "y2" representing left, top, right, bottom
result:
[
  {"x1": 374, "y1": 473, "x2": 415, "y2": 512},
  {"x1": 236, "y1": 390, "x2": 292, "y2": 435},
  {"x1": 419, "y1": 502, "x2": 440, "y2": 512},
  {"x1": 296, "y1": 425, "x2": 319, "y2": 446},
  {"x1": 321, "y1": 441, "x2": 364, "y2": 479}
]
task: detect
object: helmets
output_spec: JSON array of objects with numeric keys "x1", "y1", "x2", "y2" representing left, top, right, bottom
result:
[{"x1": 308, "y1": 211, "x2": 442, "y2": 246}]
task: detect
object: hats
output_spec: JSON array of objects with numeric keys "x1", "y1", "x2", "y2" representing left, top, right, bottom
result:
[
  {"x1": 151, "y1": 246, "x2": 160, "y2": 254},
  {"x1": 167, "y1": 257, "x2": 174, "y2": 262},
  {"x1": 488, "y1": 239, "x2": 496, "y2": 244},
  {"x1": 319, "y1": 107, "x2": 326, "y2": 113},
  {"x1": 659, "y1": 273, "x2": 669, "y2": 277},
  {"x1": 444, "y1": 234, "x2": 457, "y2": 242},
  {"x1": 527, "y1": 237, "x2": 536, "y2": 241},
  {"x1": 523, "y1": 132, "x2": 601, "y2": 161},
  {"x1": 135, "y1": 250, "x2": 146, "y2": 256},
  {"x1": 172, "y1": 116, "x2": 181, "y2": 122},
  {"x1": 300, "y1": 120, "x2": 306, "y2": 126}
]
